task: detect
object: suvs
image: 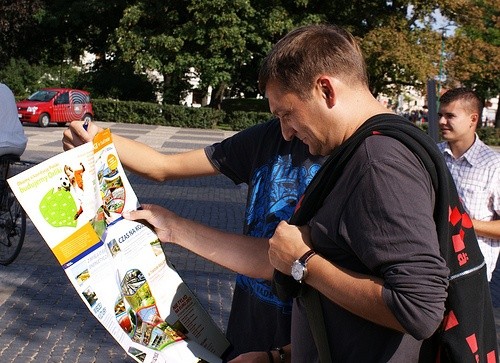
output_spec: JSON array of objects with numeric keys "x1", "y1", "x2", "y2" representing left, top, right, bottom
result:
[{"x1": 17, "y1": 89, "x2": 94, "y2": 127}]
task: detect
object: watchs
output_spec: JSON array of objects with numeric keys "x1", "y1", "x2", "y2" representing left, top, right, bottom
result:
[{"x1": 291, "y1": 250, "x2": 316, "y2": 284}]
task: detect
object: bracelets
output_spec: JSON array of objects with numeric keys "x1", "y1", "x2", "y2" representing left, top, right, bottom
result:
[
  {"x1": 274, "y1": 346, "x2": 286, "y2": 363},
  {"x1": 266, "y1": 350, "x2": 274, "y2": 362}
]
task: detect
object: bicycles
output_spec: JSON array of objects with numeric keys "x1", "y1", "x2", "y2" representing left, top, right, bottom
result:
[{"x1": 0, "y1": 153, "x2": 26, "y2": 266}]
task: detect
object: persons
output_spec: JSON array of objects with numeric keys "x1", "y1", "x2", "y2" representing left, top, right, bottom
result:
[
  {"x1": 0, "y1": 83, "x2": 27, "y2": 210},
  {"x1": 63, "y1": 162, "x2": 85, "y2": 213},
  {"x1": 63, "y1": 117, "x2": 332, "y2": 363},
  {"x1": 122, "y1": 25, "x2": 451, "y2": 363},
  {"x1": 436, "y1": 88, "x2": 500, "y2": 308},
  {"x1": 386, "y1": 101, "x2": 428, "y2": 123}
]
